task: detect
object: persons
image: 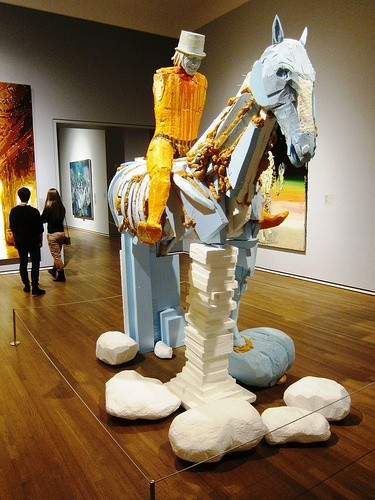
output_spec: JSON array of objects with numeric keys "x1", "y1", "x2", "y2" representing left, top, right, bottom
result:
[
  {"x1": 9, "y1": 187, "x2": 46, "y2": 295},
  {"x1": 136, "y1": 31, "x2": 289, "y2": 244},
  {"x1": 40, "y1": 188, "x2": 66, "y2": 282}
]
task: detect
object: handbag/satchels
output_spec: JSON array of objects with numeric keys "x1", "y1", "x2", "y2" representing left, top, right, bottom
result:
[{"x1": 64, "y1": 236, "x2": 71, "y2": 245}]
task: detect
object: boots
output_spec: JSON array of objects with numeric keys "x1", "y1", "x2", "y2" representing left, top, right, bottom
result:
[
  {"x1": 48, "y1": 267, "x2": 58, "y2": 279},
  {"x1": 53, "y1": 270, "x2": 66, "y2": 282}
]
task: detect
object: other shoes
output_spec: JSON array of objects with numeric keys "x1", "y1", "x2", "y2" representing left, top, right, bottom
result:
[
  {"x1": 23, "y1": 285, "x2": 30, "y2": 293},
  {"x1": 32, "y1": 286, "x2": 45, "y2": 295}
]
